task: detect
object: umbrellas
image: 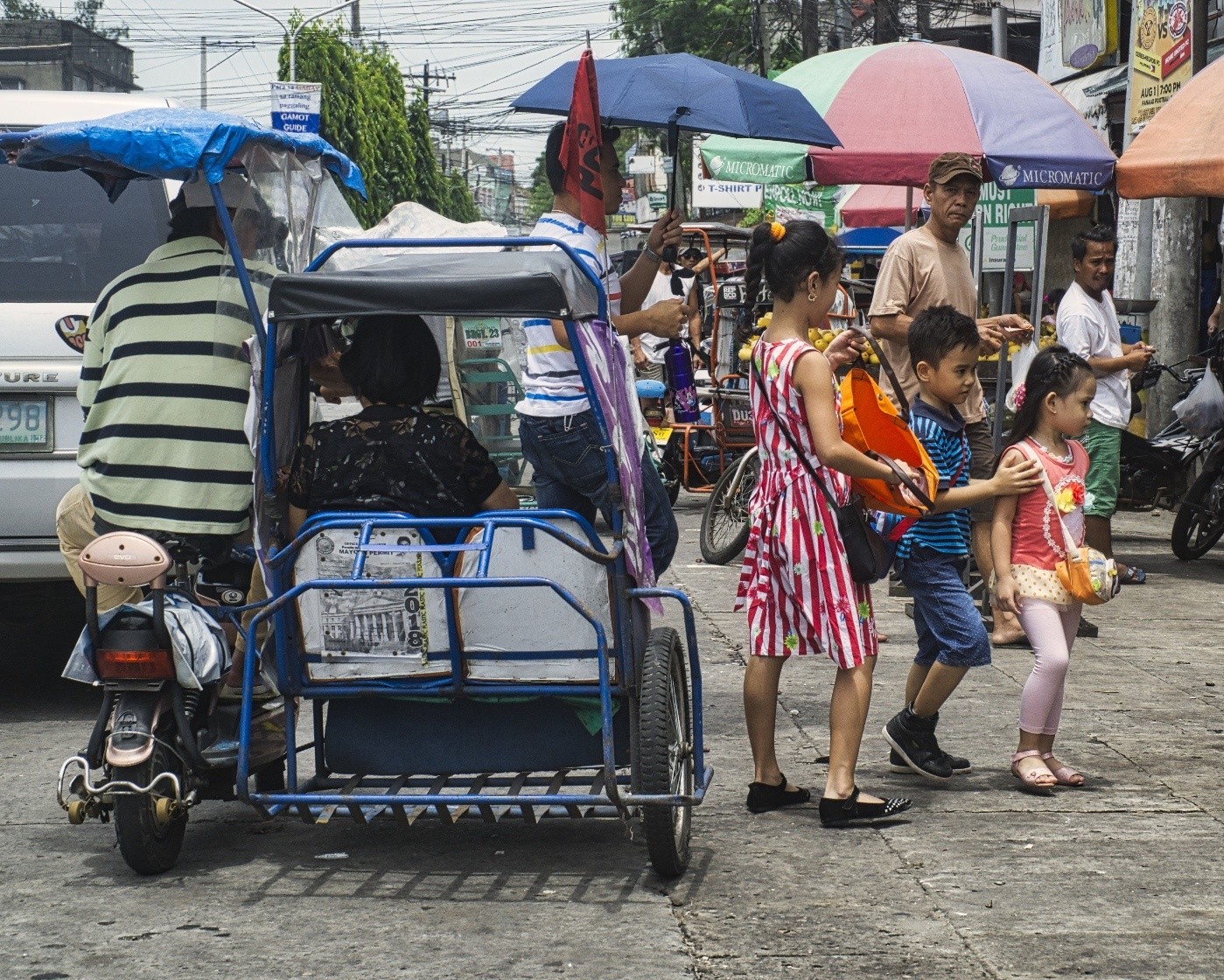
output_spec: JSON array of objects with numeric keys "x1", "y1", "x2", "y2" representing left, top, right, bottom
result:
[
  {"x1": 509, "y1": 49, "x2": 846, "y2": 268},
  {"x1": 1114, "y1": 53, "x2": 1224, "y2": 202},
  {"x1": 698, "y1": 33, "x2": 1119, "y2": 232}
]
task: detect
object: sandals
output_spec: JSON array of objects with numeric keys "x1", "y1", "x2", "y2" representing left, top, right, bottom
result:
[
  {"x1": 1011, "y1": 750, "x2": 1055, "y2": 788},
  {"x1": 1041, "y1": 752, "x2": 1085, "y2": 786},
  {"x1": 1119, "y1": 566, "x2": 1146, "y2": 585}
]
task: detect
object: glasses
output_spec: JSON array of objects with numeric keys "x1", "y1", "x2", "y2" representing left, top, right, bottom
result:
[{"x1": 679, "y1": 253, "x2": 700, "y2": 259}]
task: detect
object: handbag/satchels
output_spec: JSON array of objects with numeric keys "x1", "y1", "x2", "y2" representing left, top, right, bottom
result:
[
  {"x1": 1171, "y1": 358, "x2": 1224, "y2": 440},
  {"x1": 837, "y1": 494, "x2": 898, "y2": 582},
  {"x1": 1005, "y1": 338, "x2": 1039, "y2": 413},
  {"x1": 1055, "y1": 546, "x2": 1120, "y2": 606}
]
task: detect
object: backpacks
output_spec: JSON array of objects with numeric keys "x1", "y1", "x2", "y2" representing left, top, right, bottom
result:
[{"x1": 840, "y1": 324, "x2": 940, "y2": 518}]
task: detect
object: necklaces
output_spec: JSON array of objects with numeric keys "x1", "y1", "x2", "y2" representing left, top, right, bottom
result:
[
  {"x1": 1026, "y1": 434, "x2": 1073, "y2": 463},
  {"x1": 551, "y1": 209, "x2": 568, "y2": 214}
]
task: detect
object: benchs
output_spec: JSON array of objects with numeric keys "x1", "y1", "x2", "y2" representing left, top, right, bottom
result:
[
  {"x1": 456, "y1": 358, "x2": 527, "y2": 459},
  {"x1": 714, "y1": 374, "x2": 757, "y2": 447},
  {"x1": 282, "y1": 509, "x2": 646, "y2": 690}
]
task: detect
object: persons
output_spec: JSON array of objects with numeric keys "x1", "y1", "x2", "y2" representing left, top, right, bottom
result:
[
  {"x1": 729, "y1": 220, "x2": 921, "y2": 825},
  {"x1": 881, "y1": 304, "x2": 1044, "y2": 781},
  {"x1": 1042, "y1": 287, "x2": 1065, "y2": 325},
  {"x1": 1012, "y1": 270, "x2": 1032, "y2": 297},
  {"x1": 1198, "y1": 221, "x2": 1221, "y2": 356},
  {"x1": 514, "y1": 117, "x2": 680, "y2": 581},
  {"x1": 866, "y1": 152, "x2": 1045, "y2": 645},
  {"x1": 54, "y1": 168, "x2": 289, "y2": 702},
  {"x1": 628, "y1": 244, "x2": 730, "y2": 413},
  {"x1": 860, "y1": 264, "x2": 879, "y2": 279},
  {"x1": 287, "y1": 312, "x2": 520, "y2": 556},
  {"x1": 989, "y1": 346, "x2": 1118, "y2": 788},
  {"x1": 1054, "y1": 219, "x2": 1157, "y2": 583}
]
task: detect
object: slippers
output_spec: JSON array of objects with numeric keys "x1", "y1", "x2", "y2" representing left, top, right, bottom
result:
[
  {"x1": 222, "y1": 672, "x2": 269, "y2": 696},
  {"x1": 992, "y1": 634, "x2": 1033, "y2": 650}
]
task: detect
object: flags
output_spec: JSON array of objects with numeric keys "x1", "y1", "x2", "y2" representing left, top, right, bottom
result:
[{"x1": 555, "y1": 48, "x2": 609, "y2": 241}]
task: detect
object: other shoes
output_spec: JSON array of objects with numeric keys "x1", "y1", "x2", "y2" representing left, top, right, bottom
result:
[
  {"x1": 747, "y1": 772, "x2": 811, "y2": 812},
  {"x1": 819, "y1": 785, "x2": 913, "y2": 824}
]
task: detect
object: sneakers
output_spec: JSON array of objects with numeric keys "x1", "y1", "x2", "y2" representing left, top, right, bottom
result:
[
  {"x1": 889, "y1": 734, "x2": 972, "y2": 774},
  {"x1": 881, "y1": 701, "x2": 953, "y2": 783}
]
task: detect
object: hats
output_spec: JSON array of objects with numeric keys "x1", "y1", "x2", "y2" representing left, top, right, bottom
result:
[
  {"x1": 929, "y1": 152, "x2": 983, "y2": 184},
  {"x1": 168, "y1": 167, "x2": 289, "y2": 249},
  {"x1": 677, "y1": 246, "x2": 703, "y2": 263}
]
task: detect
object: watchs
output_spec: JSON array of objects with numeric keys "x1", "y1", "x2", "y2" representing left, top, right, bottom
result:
[{"x1": 643, "y1": 242, "x2": 663, "y2": 264}]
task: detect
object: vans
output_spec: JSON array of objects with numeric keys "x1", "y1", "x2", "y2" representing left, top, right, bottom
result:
[{"x1": 0, "y1": 81, "x2": 183, "y2": 593}]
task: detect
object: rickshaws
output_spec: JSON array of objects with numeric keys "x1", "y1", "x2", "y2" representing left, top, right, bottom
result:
[
  {"x1": 1, "y1": 106, "x2": 716, "y2": 881},
  {"x1": 458, "y1": 217, "x2": 1224, "y2": 566}
]
